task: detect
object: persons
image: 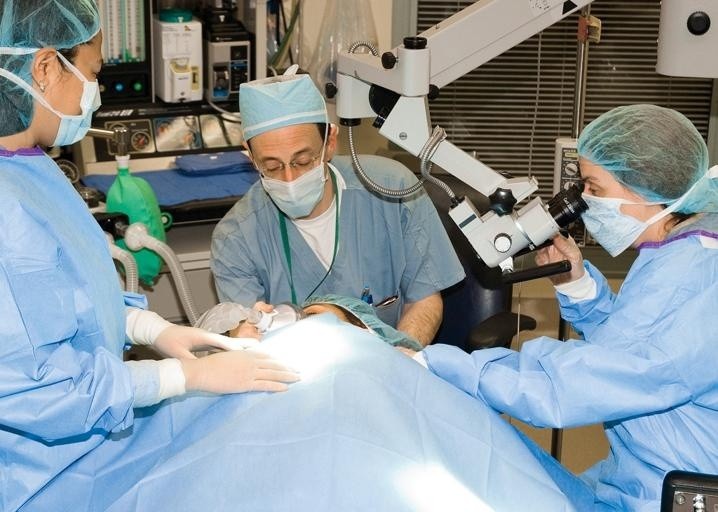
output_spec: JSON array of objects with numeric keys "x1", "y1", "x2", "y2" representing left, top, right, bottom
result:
[
  {"x1": 384, "y1": 98, "x2": 718, "y2": 512},
  {"x1": 1, "y1": 0, "x2": 301, "y2": 509},
  {"x1": 294, "y1": 289, "x2": 391, "y2": 341},
  {"x1": 208, "y1": 71, "x2": 469, "y2": 354}
]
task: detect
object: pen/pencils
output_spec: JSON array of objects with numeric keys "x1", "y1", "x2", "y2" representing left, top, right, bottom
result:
[{"x1": 361, "y1": 285, "x2": 399, "y2": 309}]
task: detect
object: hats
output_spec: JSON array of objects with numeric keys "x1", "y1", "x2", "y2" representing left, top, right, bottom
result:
[{"x1": 239, "y1": 74, "x2": 330, "y2": 141}]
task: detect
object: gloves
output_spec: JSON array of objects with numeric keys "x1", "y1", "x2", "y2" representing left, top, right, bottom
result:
[
  {"x1": 123, "y1": 306, "x2": 259, "y2": 360},
  {"x1": 126, "y1": 351, "x2": 302, "y2": 409}
]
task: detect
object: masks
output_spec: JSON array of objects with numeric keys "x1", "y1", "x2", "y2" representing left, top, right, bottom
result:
[
  {"x1": 581, "y1": 193, "x2": 668, "y2": 257},
  {"x1": 32, "y1": 51, "x2": 102, "y2": 147},
  {"x1": 253, "y1": 124, "x2": 328, "y2": 220}
]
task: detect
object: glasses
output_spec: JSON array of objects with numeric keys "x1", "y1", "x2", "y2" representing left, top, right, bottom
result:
[{"x1": 263, "y1": 144, "x2": 323, "y2": 177}]
